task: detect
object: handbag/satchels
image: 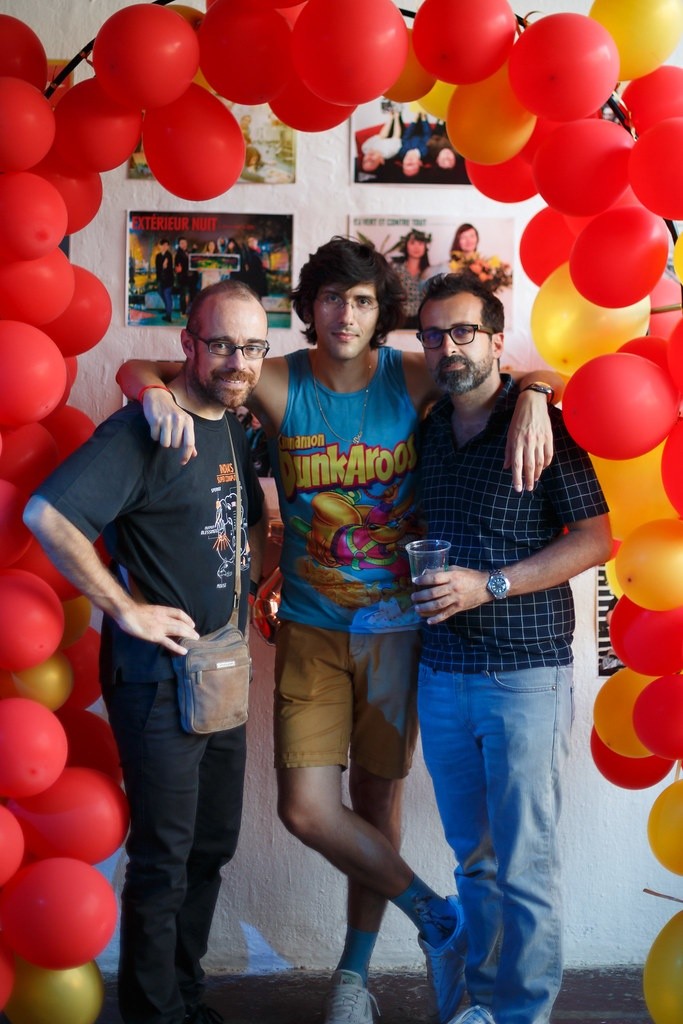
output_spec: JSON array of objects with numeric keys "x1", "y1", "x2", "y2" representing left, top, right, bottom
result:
[{"x1": 171, "y1": 622, "x2": 250, "y2": 735}]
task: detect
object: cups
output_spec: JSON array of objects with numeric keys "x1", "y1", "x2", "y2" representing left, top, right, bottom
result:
[{"x1": 405, "y1": 539, "x2": 451, "y2": 617}]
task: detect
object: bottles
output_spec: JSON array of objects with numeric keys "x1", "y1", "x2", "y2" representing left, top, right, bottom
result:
[{"x1": 261, "y1": 521, "x2": 284, "y2": 578}]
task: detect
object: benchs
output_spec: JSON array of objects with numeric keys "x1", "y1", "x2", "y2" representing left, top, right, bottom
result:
[{"x1": 354, "y1": 123, "x2": 435, "y2": 181}]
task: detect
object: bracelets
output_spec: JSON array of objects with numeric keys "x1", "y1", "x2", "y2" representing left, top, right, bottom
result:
[{"x1": 137, "y1": 384, "x2": 176, "y2": 403}]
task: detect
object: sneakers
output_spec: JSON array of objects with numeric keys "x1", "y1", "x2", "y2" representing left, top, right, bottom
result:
[
  {"x1": 417, "y1": 894, "x2": 467, "y2": 1023},
  {"x1": 325, "y1": 969, "x2": 381, "y2": 1024},
  {"x1": 447, "y1": 1004, "x2": 495, "y2": 1024}
]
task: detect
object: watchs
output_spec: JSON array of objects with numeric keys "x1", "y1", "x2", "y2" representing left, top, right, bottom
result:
[
  {"x1": 520, "y1": 381, "x2": 555, "y2": 404},
  {"x1": 486, "y1": 568, "x2": 509, "y2": 599}
]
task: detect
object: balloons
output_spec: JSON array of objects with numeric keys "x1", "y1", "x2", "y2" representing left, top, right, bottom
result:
[{"x1": 1, "y1": 0, "x2": 682, "y2": 1023}]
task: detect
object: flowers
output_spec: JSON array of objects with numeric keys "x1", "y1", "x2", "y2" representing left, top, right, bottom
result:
[{"x1": 456, "y1": 254, "x2": 513, "y2": 294}]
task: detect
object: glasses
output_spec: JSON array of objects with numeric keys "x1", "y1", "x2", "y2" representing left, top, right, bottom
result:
[
  {"x1": 314, "y1": 295, "x2": 380, "y2": 314},
  {"x1": 415, "y1": 321, "x2": 493, "y2": 350},
  {"x1": 185, "y1": 332, "x2": 271, "y2": 361}
]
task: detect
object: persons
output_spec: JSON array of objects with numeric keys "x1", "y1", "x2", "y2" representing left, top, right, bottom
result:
[
  {"x1": 156, "y1": 237, "x2": 271, "y2": 321},
  {"x1": 449, "y1": 220, "x2": 480, "y2": 261},
  {"x1": 358, "y1": 105, "x2": 458, "y2": 181},
  {"x1": 21, "y1": 277, "x2": 274, "y2": 1023},
  {"x1": 409, "y1": 271, "x2": 614, "y2": 1024},
  {"x1": 109, "y1": 229, "x2": 555, "y2": 1023},
  {"x1": 387, "y1": 228, "x2": 433, "y2": 325}
]
